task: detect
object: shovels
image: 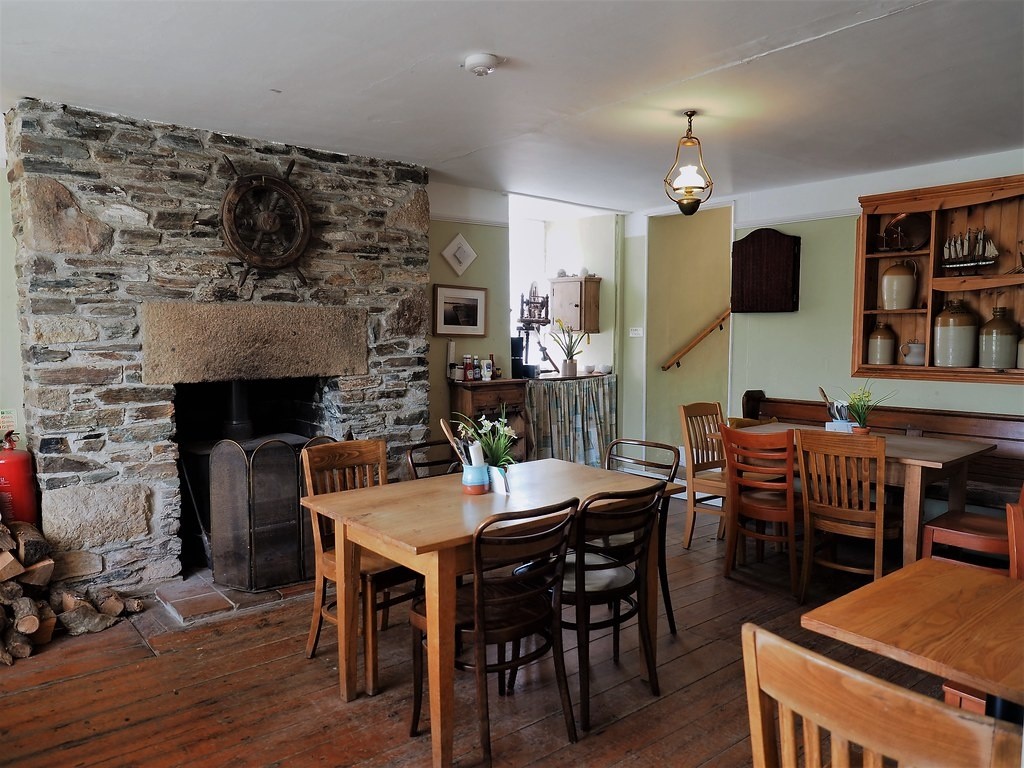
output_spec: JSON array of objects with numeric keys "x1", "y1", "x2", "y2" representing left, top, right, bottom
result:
[{"x1": 819, "y1": 387, "x2": 838, "y2": 420}]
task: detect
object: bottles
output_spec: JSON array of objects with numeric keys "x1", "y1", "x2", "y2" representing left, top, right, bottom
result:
[
  {"x1": 455, "y1": 366, "x2": 464, "y2": 382},
  {"x1": 449, "y1": 363, "x2": 459, "y2": 379},
  {"x1": 489, "y1": 353, "x2": 496, "y2": 380},
  {"x1": 462, "y1": 354, "x2": 466, "y2": 368},
  {"x1": 867, "y1": 322, "x2": 894, "y2": 364},
  {"x1": 1018, "y1": 337, "x2": 1024, "y2": 369},
  {"x1": 934, "y1": 299, "x2": 977, "y2": 367},
  {"x1": 465, "y1": 354, "x2": 474, "y2": 381},
  {"x1": 496, "y1": 367, "x2": 501, "y2": 378},
  {"x1": 978, "y1": 307, "x2": 1018, "y2": 369},
  {"x1": 481, "y1": 360, "x2": 493, "y2": 381},
  {"x1": 473, "y1": 356, "x2": 481, "y2": 381},
  {"x1": 881, "y1": 258, "x2": 917, "y2": 310}
]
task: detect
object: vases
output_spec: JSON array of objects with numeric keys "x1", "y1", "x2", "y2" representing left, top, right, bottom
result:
[
  {"x1": 562, "y1": 356, "x2": 578, "y2": 376},
  {"x1": 851, "y1": 426, "x2": 872, "y2": 435},
  {"x1": 487, "y1": 465, "x2": 508, "y2": 494}
]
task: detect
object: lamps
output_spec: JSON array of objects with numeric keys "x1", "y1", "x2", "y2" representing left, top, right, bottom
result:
[{"x1": 664, "y1": 109, "x2": 713, "y2": 215}]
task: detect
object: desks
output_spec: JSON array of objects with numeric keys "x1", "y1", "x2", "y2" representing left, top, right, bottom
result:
[
  {"x1": 300, "y1": 457, "x2": 686, "y2": 768},
  {"x1": 706, "y1": 422, "x2": 997, "y2": 568},
  {"x1": 801, "y1": 557, "x2": 1024, "y2": 704},
  {"x1": 539, "y1": 372, "x2": 606, "y2": 381}
]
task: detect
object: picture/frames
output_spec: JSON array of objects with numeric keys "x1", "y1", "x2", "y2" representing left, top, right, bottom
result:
[{"x1": 432, "y1": 283, "x2": 489, "y2": 338}]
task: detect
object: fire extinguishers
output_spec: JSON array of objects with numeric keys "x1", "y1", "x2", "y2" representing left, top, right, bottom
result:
[{"x1": 0, "y1": 430, "x2": 38, "y2": 524}]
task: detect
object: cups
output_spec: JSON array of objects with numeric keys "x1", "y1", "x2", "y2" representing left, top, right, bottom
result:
[
  {"x1": 601, "y1": 366, "x2": 611, "y2": 374},
  {"x1": 585, "y1": 366, "x2": 594, "y2": 374}
]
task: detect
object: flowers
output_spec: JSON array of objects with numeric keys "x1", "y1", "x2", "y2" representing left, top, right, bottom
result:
[
  {"x1": 450, "y1": 402, "x2": 518, "y2": 469},
  {"x1": 549, "y1": 318, "x2": 590, "y2": 359},
  {"x1": 828, "y1": 376, "x2": 896, "y2": 426}
]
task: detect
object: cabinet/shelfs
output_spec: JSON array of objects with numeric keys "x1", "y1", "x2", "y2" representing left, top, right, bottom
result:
[
  {"x1": 848, "y1": 174, "x2": 1024, "y2": 385},
  {"x1": 547, "y1": 278, "x2": 602, "y2": 334},
  {"x1": 449, "y1": 379, "x2": 529, "y2": 464}
]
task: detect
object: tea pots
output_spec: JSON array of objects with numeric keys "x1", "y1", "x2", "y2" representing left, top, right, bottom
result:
[{"x1": 900, "y1": 339, "x2": 926, "y2": 366}]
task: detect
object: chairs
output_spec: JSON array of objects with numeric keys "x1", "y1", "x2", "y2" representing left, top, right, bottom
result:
[{"x1": 302, "y1": 403, "x2": 1024, "y2": 768}]
